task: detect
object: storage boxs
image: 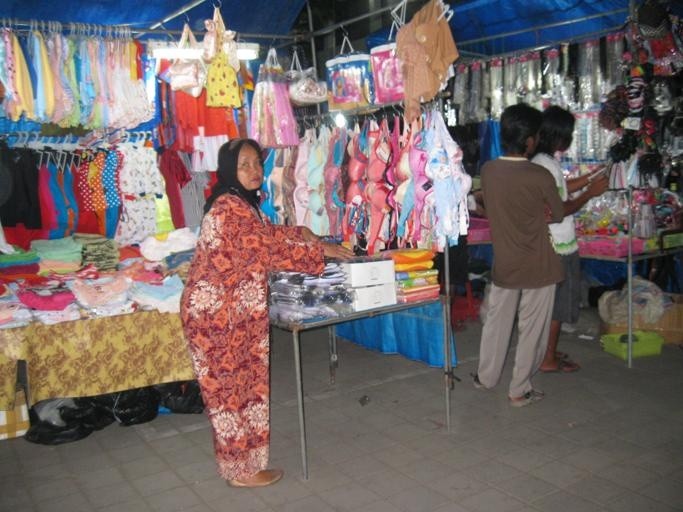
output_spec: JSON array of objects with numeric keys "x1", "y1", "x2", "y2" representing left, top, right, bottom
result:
[
  {"x1": 342, "y1": 282, "x2": 397, "y2": 312},
  {"x1": 336, "y1": 256, "x2": 394, "y2": 285},
  {"x1": 2, "y1": 382, "x2": 32, "y2": 443}
]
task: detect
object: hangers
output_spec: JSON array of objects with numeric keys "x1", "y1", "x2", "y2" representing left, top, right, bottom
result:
[
  {"x1": 4, "y1": 126, "x2": 151, "y2": 176},
  {"x1": 0, "y1": 12, "x2": 137, "y2": 41},
  {"x1": 294, "y1": 92, "x2": 442, "y2": 141}
]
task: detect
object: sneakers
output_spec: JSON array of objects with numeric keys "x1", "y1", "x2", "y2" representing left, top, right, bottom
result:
[
  {"x1": 470, "y1": 374, "x2": 491, "y2": 393},
  {"x1": 226, "y1": 469, "x2": 285, "y2": 489},
  {"x1": 506, "y1": 388, "x2": 546, "y2": 408}
]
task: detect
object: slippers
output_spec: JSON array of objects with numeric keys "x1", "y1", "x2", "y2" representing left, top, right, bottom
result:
[{"x1": 540, "y1": 358, "x2": 580, "y2": 374}]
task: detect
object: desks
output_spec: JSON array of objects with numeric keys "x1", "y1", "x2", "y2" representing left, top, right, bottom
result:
[
  {"x1": 575, "y1": 251, "x2": 682, "y2": 371},
  {"x1": 261, "y1": 291, "x2": 455, "y2": 479},
  {"x1": 0, "y1": 262, "x2": 198, "y2": 443}
]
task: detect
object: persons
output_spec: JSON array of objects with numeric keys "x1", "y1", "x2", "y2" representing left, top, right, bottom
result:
[
  {"x1": 181, "y1": 138, "x2": 356, "y2": 489},
  {"x1": 473, "y1": 102, "x2": 566, "y2": 406},
  {"x1": 530, "y1": 106, "x2": 609, "y2": 372}
]
